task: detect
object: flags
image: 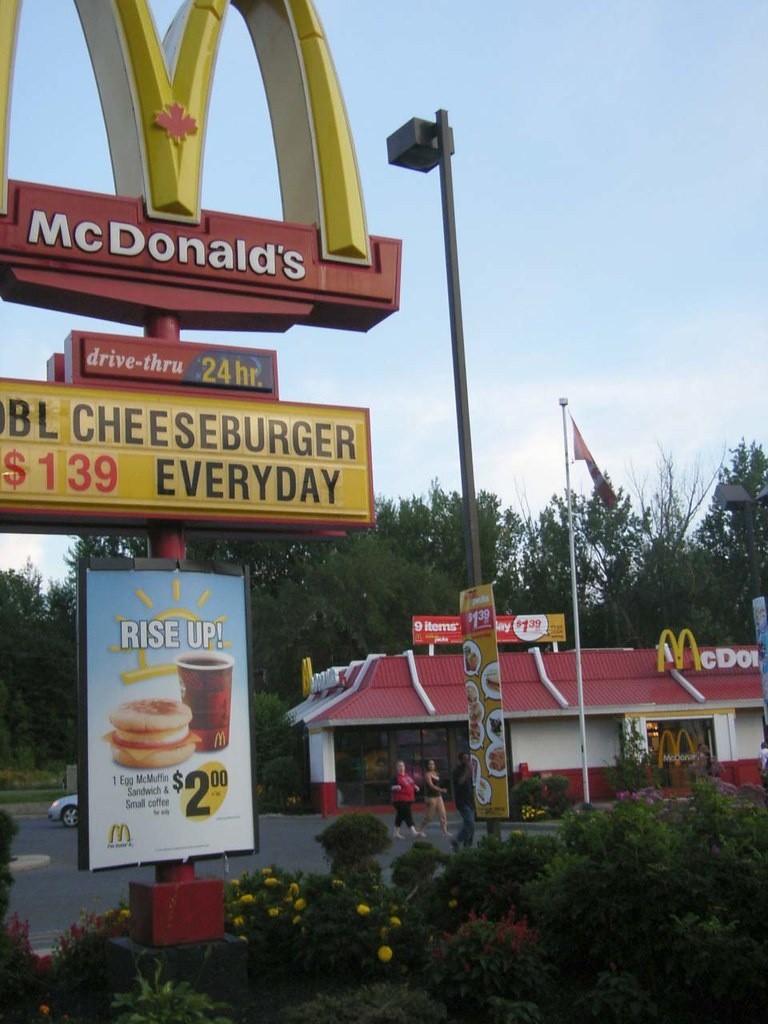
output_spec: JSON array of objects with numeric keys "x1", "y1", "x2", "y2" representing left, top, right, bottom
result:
[{"x1": 570, "y1": 413, "x2": 617, "y2": 509}]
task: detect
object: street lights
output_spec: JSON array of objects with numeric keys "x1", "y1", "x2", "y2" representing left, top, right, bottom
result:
[
  {"x1": 711, "y1": 481, "x2": 767, "y2": 804},
  {"x1": 385, "y1": 109, "x2": 505, "y2": 845}
]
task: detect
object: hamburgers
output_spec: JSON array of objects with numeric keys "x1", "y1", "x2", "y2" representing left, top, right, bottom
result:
[{"x1": 104, "y1": 700, "x2": 201, "y2": 767}]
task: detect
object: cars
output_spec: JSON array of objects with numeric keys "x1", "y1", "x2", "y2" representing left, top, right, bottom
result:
[{"x1": 45, "y1": 793, "x2": 78, "y2": 828}]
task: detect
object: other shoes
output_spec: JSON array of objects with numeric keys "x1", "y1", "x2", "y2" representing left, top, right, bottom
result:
[
  {"x1": 418, "y1": 833, "x2": 427, "y2": 838},
  {"x1": 393, "y1": 834, "x2": 405, "y2": 839},
  {"x1": 441, "y1": 832, "x2": 453, "y2": 838},
  {"x1": 408, "y1": 828, "x2": 419, "y2": 837}
]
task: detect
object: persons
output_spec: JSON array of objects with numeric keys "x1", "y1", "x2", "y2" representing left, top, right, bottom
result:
[
  {"x1": 450, "y1": 753, "x2": 479, "y2": 853},
  {"x1": 756, "y1": 742, "x2": 768, "y2": 788},
  {"x1": 388, "y1": 760, "x2": 422, "y2": 840},
  {"x1": 422, "y1": 758, "x2": 453, "y2": 838}
]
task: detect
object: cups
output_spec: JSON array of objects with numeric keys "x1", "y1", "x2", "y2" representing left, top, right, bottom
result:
[{"x1": 174, "y1": 652, "x2": 234, "y2": 750}]
